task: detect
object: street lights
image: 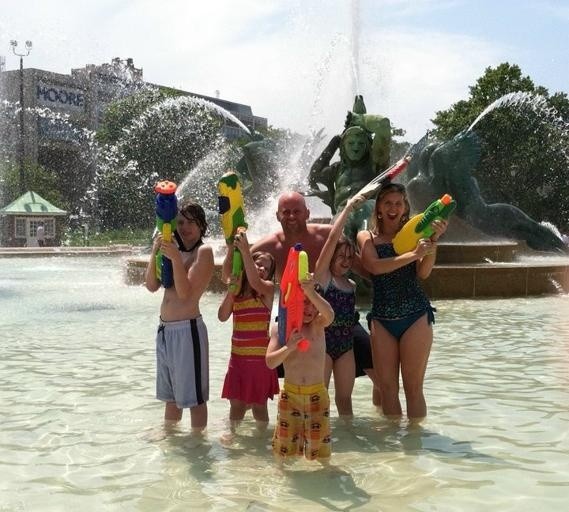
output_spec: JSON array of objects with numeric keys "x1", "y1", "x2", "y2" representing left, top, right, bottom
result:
[{"x1": 6, "y1": 39, "x2": 34, "y2": 192}]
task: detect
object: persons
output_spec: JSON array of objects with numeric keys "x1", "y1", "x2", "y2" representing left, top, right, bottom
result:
[
  {"x1": 145, "y1": 202, "x2": 214, "y2": 427},
  {"x1": 36, "y1": 220, "x2": 45, "y2": 247},
  {"x1": 315, "y1": 196, "x2": 368, "y2": 420},
  {"x1": 307, "y1": 111, "x2": 391, "y2": 243},
  {"x1": 265, "y1": 272, "x2": 335, "y2": 461},
  {"x1": 217, "y1": 231, "x2": 277, "y2": 424},
  {"x1": 360, "y1": 182, "x2": 449, "y2": 418},
  {"x1": 221, "y1": 191, "x2": 383, "y2": 406}
]
